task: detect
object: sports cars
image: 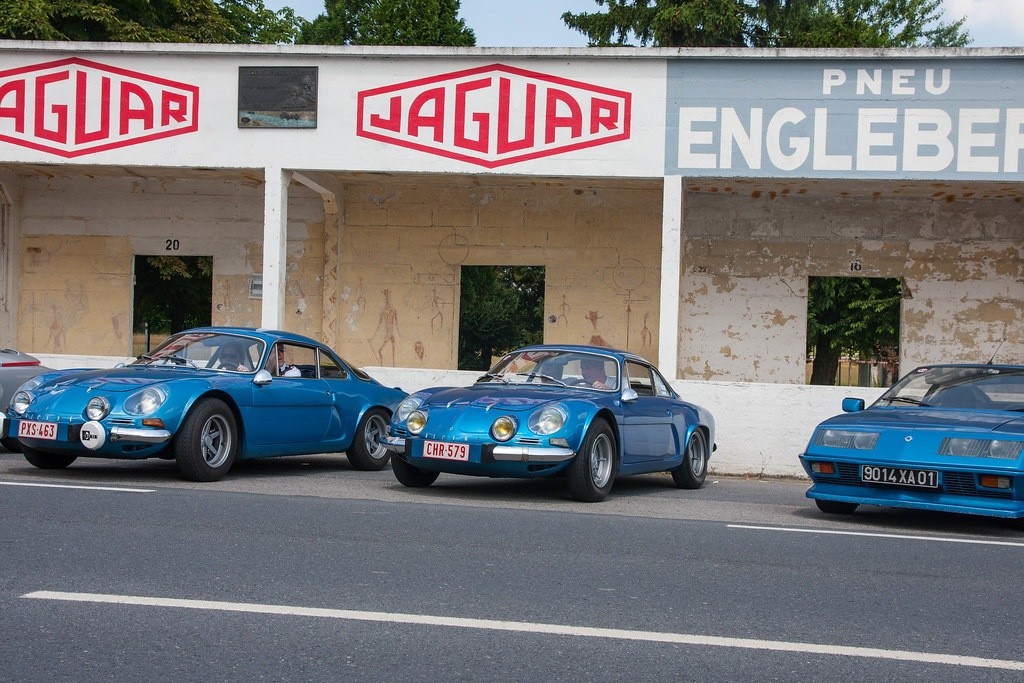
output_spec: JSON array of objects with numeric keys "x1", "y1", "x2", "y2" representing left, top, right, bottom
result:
[
  {"x1": 378, "y1": 343, "x2": 718, "y2": 503},
  {"x1": 798, "y1": 363, "x2": 1024, "y2": 529},
  {"x1": 8, "y1": 325, "x2": 410, "y2": 483}
]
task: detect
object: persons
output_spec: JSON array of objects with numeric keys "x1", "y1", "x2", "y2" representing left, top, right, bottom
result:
[
  {"x1": 880, "y1": 357, "x2": 898, "y2": 387},
  {"x1": 236, "y1": 341, "x2": 301, "y2": 378},
  {"x1": 541, "y1": 363, "x2": 563, "y2": 383},
  {"x1": 580, "y1": 359, "x2": 629, "y2": 392},
  {"x1": 219, "y1": 342, "x2": 244, "y2": 371}
]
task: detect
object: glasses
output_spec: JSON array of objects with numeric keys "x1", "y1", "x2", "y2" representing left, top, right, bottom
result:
[
  {"x1": 277, "y1": 348, "x2": 286, "y2": 352},
  {"x1": 222, "y1": 353, "x2": 240, "y2": 359}
]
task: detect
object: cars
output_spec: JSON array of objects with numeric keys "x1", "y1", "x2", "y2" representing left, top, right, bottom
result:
[{"x1": 0, "y1": 348, "x2": 57, "y2": 453}]
